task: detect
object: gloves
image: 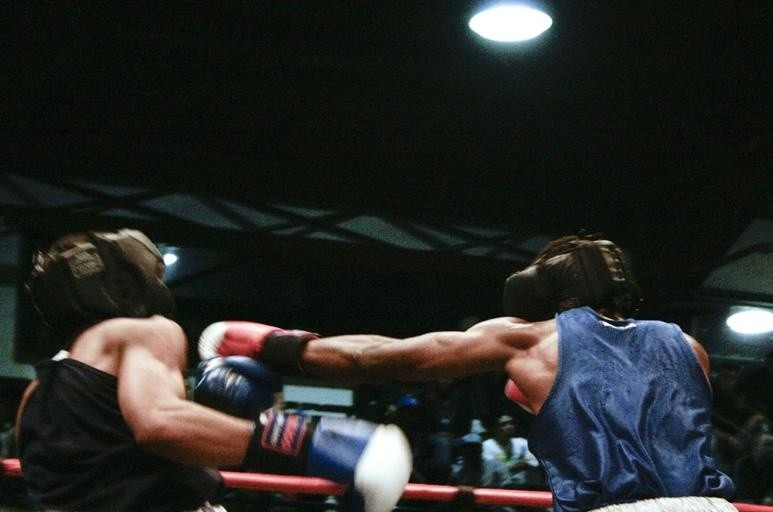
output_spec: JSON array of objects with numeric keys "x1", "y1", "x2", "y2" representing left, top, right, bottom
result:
[
  {"x1": 193, "y1": 320, "x2": 318, "y2": 414},
  {"x1": 259, "y1": 408, "x2": 415, "y2": 512}
]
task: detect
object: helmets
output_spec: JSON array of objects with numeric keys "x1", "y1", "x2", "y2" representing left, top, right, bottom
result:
[
  {"x1": 23, "y1": 226, "x2": 178, "y2": 333},
  {"x1": 500, "y1": 239, "x2": 643, "y2": 319}
]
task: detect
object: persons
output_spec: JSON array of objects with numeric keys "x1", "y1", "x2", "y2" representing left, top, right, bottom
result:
[
  {"x1": 14, "y1": 229, "x2": 416, "y2": 511},
  {"x1": 711, "y1": 355, "x2": 770, "y2": 505},
  {"x1": 354, "y1": 385, "x2": 544, "y2": 489},
  {"x1": 196, "y1": 229, "x2": 740, "y2": 512}
]
